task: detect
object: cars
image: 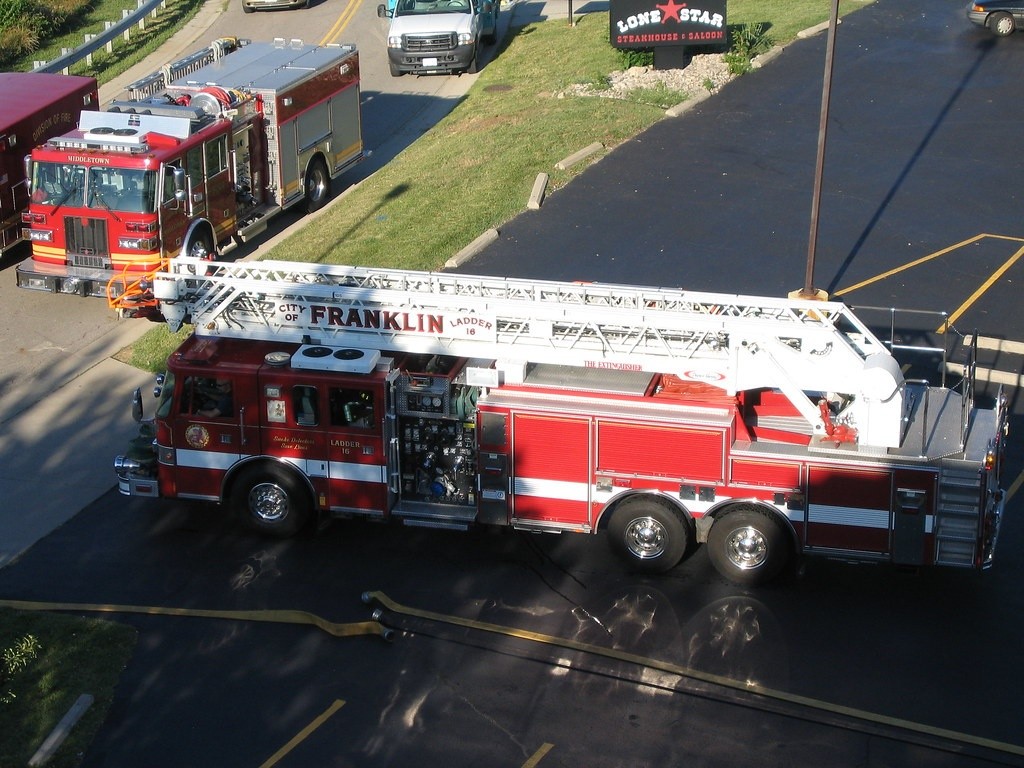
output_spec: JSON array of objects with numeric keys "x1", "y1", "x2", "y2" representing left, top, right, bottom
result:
[
  {"x1": 242, "y1": 0, "x2": 311, "y2": 14},
  {"x1": 965, "y1": 0, "x2": 1024, "y2": 37}
]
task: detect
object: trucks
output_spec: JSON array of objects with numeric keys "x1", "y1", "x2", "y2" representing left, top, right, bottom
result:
[
  {"x1": 377, "y1": 0, "x2": 501, "y2": 77},
  {"x1": 0, "y1": 71, "x2": 100, "y2": 258}
]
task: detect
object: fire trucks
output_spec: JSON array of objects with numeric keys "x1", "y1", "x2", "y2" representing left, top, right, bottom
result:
[
  {"x1": 14, "y1": 34, "x2": 372, "y2": 317},
  {"x1": 115, "y1": 257, "x2": 1009, "y2": 590}
]
